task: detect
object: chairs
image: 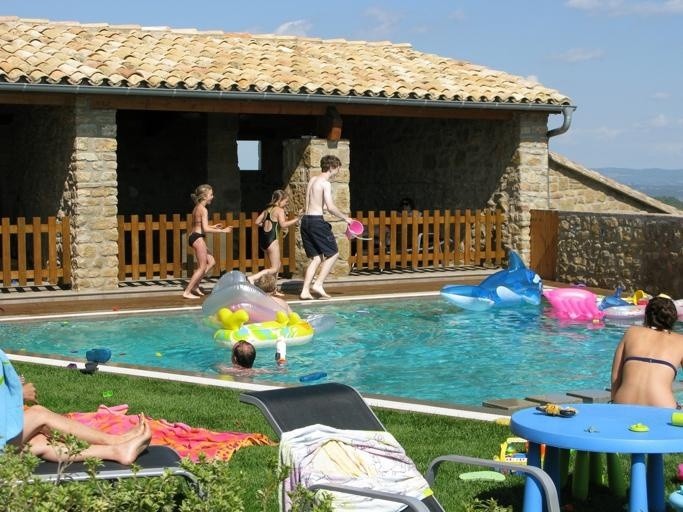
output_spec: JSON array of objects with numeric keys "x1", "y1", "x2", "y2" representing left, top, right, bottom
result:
[
  {"x1": 240, "y1": 383, "x2": 560, "y2": 511},
  {"x1": 28, "y1": 444, "x2": 203, "y2": 494}
]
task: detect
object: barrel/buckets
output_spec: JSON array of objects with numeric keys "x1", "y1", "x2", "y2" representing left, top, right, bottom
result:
[{"x1": 346, "y1": 221, "x2": 365, "y2": 241}]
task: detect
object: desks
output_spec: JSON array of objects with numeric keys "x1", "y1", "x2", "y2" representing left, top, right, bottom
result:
[{"x1": 510, "y1": 403, "x2": 683, "y2": 512}]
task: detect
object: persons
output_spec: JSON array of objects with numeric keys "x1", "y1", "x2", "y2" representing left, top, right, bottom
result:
[
  {"x1": 217, "y1": 340, "x2": 286, "y2": 376},
  {"x1": 183, "y1": 184, "x2": 233, "y2": 299},
  {"x1": 610, "y1": 298, "x2": 683, "y2": 408},
  {"x1": 0, "y1": 349, "x2": 151, "y2": 466},
  {"x1": 247, "y1": 190, "x2": 304, "y2": 297},
  {"x1": 300, "y1": 155, "x2": 352, "y2": 300},
  {"x1": 397, "y1": 198, "x2": 415, "y2": 245}
]
